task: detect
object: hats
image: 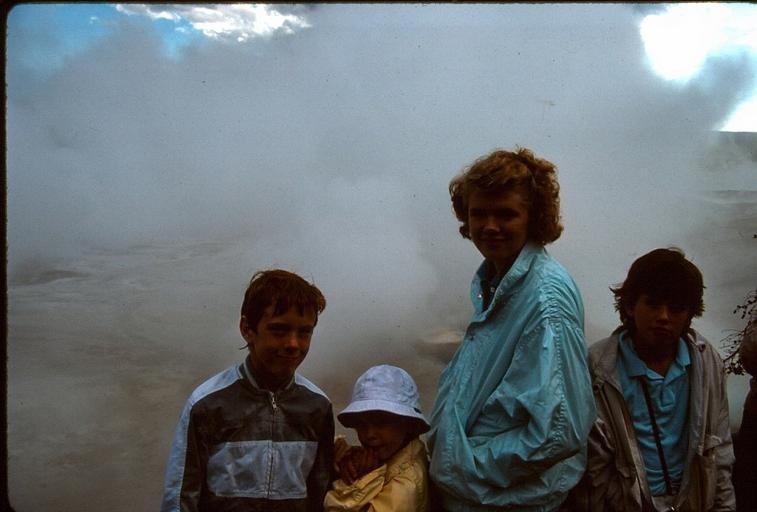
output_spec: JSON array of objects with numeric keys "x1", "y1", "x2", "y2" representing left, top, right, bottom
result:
[{"x1": 336, "y1": 364, "x2": 432, "y2": 435}]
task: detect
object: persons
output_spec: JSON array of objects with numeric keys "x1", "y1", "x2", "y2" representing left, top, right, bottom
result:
[
  {"x1": 424, "y1": 147, "x2": 594, "y2": 511},
  {"x1": 159, "y1": 268, "x2": 334, "y2": 510},
  {"x1": 573, "y1": 246, "x2": 738, "y2": 511},
  {"x1": 318, "y1": 362, "x2": 432, "y2": 511}
]
